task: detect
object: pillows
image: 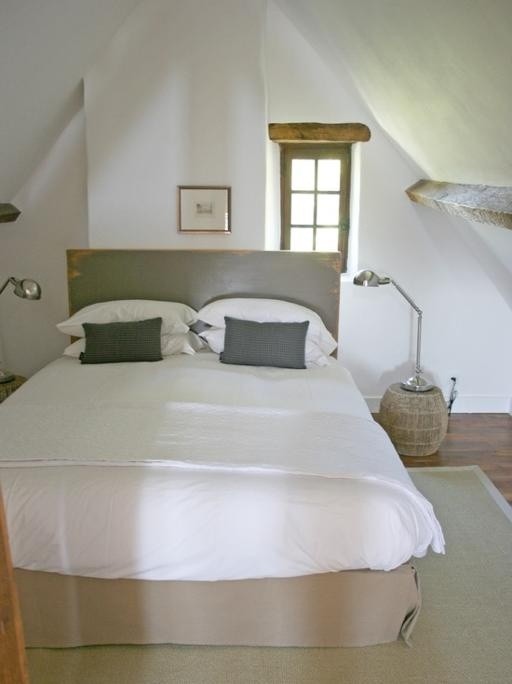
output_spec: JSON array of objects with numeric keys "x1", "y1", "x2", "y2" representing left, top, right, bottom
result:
[
  {"x1": 55, "y1": 300, "x2": 196, "y2": 338},
  {"x1": 78, "y1": 316, "x2": 163, "y2": 364},
  {"x1": 194, "y1": 297, "x2": 340, "y2": 354},
  {"x1": 220, "y1": 316, "x2": 310, "y2": 369},
  {"x1": 62, "y1": 330, "x2": 208, "y2": 359},
  {"x1": 198, "y1": 327, "x2": 328, "y2": 366}
]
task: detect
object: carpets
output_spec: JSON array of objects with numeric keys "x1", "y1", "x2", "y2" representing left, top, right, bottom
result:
[{"x1": 13, "y1": 464, "x2": 511, "y2": 682}]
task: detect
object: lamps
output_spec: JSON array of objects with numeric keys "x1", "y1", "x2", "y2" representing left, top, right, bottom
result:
[
  {"x1": 1, "y1": 273, "x2": 43, "y2": 303},
  {"x1": 352, "y1": 269, "x2": 435, "y2": 392}
]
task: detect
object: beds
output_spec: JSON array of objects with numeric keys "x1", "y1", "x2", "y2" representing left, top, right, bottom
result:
[{"x1": 1, "y1": 249, "x2": 446, "y2": 648}]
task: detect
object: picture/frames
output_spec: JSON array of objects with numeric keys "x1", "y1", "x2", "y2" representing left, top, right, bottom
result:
[{"x1": 177, "y1": 185, "x2": 232, "y2": 235}]
